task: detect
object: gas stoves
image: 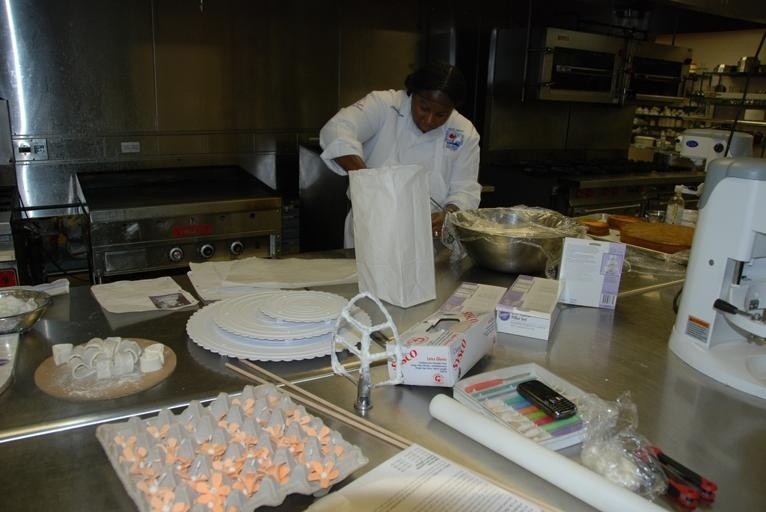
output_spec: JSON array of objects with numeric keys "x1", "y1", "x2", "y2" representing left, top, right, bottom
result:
[{"x1": 534, "y1": 157, "x2": 655, "y2": 176}]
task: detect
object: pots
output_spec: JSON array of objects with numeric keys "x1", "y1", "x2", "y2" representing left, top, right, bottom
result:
[{"x1": 714, "y1": 56, "x2": 761, "y2": 73}]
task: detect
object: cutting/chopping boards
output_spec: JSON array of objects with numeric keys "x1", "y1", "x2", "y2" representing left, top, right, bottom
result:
[{"x1": 34, "y1": 336, "x2": 177, "y2": 403}]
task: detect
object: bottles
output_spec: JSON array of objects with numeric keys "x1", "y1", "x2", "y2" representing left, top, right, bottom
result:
[
  {"x1": 632, "y1": 107, "x2": 686, "y2": 137},
  {"x1": 665, "y1": 190, "x2": 685, "y2": 226}
]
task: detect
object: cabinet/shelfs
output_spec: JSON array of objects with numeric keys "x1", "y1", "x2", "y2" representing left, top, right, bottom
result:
[{"x1": 630, "y1": 64, "x2": 766, "y2": 144}]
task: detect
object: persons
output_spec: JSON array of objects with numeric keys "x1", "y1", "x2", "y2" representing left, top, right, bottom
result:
[{"x1": 318, "y1": 61, "x2": 483, "y2": 250}]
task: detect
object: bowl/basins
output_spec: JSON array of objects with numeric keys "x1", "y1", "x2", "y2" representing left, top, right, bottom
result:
[
  {"x1": 645, "y1": 210, "x2": 665, "y2": 224},
  {"x1": 0, "y1": 290, "x2": 54, "y2": 334},
  {"x1": 451, "y1": 208, "x2": 583, "y2": 273}
]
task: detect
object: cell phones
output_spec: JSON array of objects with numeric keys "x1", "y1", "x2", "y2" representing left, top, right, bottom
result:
[{"x1": 517, "y1": 380, "x2": 577, "y2": 419}]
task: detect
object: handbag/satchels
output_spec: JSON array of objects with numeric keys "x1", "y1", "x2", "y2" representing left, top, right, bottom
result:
[{"x1": 348, "y1": 163, "x2": 438, "y2": 309}]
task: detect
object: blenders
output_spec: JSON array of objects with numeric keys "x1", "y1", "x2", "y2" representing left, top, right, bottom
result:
[{"x1": 668, "y1": 159, "x2": 766, "y2": 402}]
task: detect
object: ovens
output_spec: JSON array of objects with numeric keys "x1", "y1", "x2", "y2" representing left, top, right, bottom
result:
[
  {"x1": 529, "y1": 27, "x2": 695, "y2": 108},
  {"x1": 74, "y1": 164, "x2": 283, "y2": 282},
  {"x1": 562, "y1": 198, "x2": 706, "y2": 218}
]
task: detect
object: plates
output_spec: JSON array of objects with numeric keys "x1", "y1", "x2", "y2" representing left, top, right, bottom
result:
[{"x1": 185, "y1": 256, "x2": 280, "y2": 306}]
